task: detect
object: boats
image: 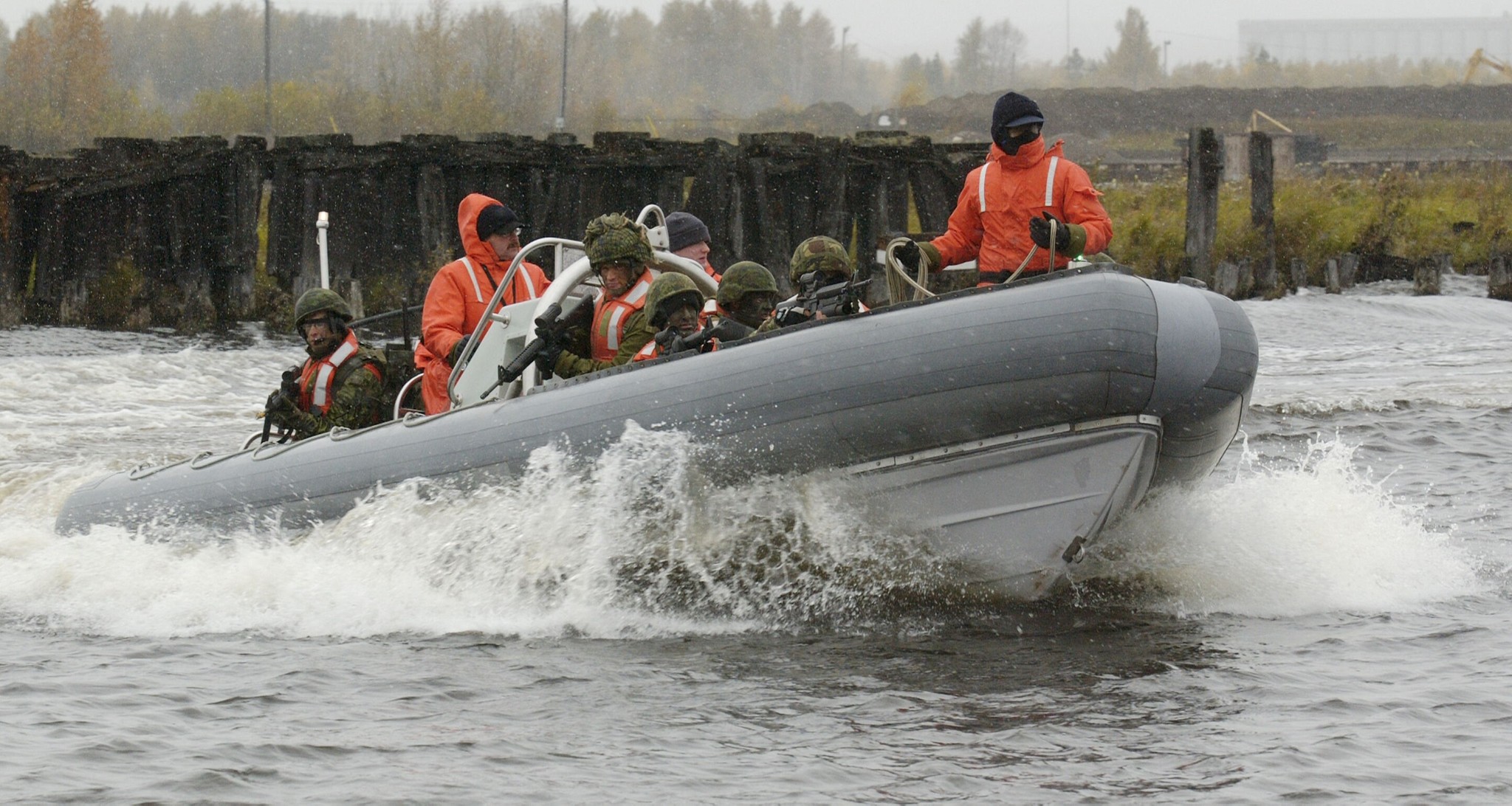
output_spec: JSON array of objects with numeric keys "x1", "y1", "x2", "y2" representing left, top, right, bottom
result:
[{"x1": 52, "y1": 235, "x2": 1259, "y2": 610}]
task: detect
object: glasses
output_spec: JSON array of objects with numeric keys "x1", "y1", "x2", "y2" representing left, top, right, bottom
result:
[{"x1": 497, "y1": 227, "x2": 523, "y2": 238}]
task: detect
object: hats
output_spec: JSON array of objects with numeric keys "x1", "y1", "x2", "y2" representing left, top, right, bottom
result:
[
  {"x1": 477, "y1": 204, "x2": 520, "y2": 241},
  {"x1": 1004, "y1": 113, "x2": 1044, "y2": 128},
  {"x1": 665, "y1": 211, "x2": 711, "y2": 250}
]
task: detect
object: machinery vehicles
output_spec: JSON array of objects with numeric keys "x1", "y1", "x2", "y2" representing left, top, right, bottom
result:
[{"x1": 1459, "y1": 48, "x2": 1512, "y2": 85}]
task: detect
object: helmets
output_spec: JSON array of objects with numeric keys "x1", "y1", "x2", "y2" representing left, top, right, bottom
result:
[
  {"x1": 583, "y1": 212, "x2": 654, "y2": 275},
  {"x1": 294, "y1": 288, "x2": 354, "y2": 327},
  {"x1": 716, "y1": 260, "x2": 778, "y2": 306},
  {"x1": 644, "y1": 271, "x2": 706, "y2": 327},
  {"x1": 790, "y1": 235, "x2": 856, "y2": 287}
]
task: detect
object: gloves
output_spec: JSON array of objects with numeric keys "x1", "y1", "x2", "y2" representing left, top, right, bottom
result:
[
  {"x1": 532, "y1": 342, "x2": 564, "y2": 373},
  {"x1": 1029, "y1": 211, "x2": 1071, "y2": 252},
  {"x1": 895, "y1": 241, "x2": 920, "y2": 271},
  {"x1": 452, "y1": 334, "x2": 480, "y2": 363},
  {"x1": 266, "y1": 398, "x2": 303, "y2": 430}
]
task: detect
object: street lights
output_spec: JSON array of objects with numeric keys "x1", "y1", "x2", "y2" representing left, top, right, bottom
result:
[{"x1": 1164, "y1": 40, "x2": 1170, "y2": 72}]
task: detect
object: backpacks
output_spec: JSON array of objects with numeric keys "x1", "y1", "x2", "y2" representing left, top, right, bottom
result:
[{"x1": 330, "y1": 342, "x2": 425, "y2": 427}]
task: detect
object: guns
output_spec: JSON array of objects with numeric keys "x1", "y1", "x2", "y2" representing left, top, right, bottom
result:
[
  {"x1": 255, "y1": 363, "x2": 302, "y2": 421},
  {"x1": 775, "y1": 266, "x2": 877, "y2": 323},
  {"x1": 652, "y1": 315, "x2": 731, "y2": 357},
  {"x1": 479, "y1": 293, "x2": 597, "y2": 400}
]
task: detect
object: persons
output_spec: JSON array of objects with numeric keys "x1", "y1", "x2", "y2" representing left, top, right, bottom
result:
[
  {"x1": 643, "y1": 212, "x2": 719, "y2": 287},
  {"x1": 534, "y1": 214, "x2": 654, "y2": 379},
  {"x1": 711, "y1": 261, "x2": 779, "y2": 342},
  {"x1": 265, "y1": 287, "x2": 386, "y2": 438},
  {"x1": 749, "y1": 234, "x2": 870, "y2": 335},
  {"x1": 421, "y1": 193, "x2": 553, "y2": 416},
  {"x1": 900, "y1": 92, "x2": 1114, "y2": 287},
  {"x1": 631, "y1": 273, "x2": 719, "y2": 361}
]
